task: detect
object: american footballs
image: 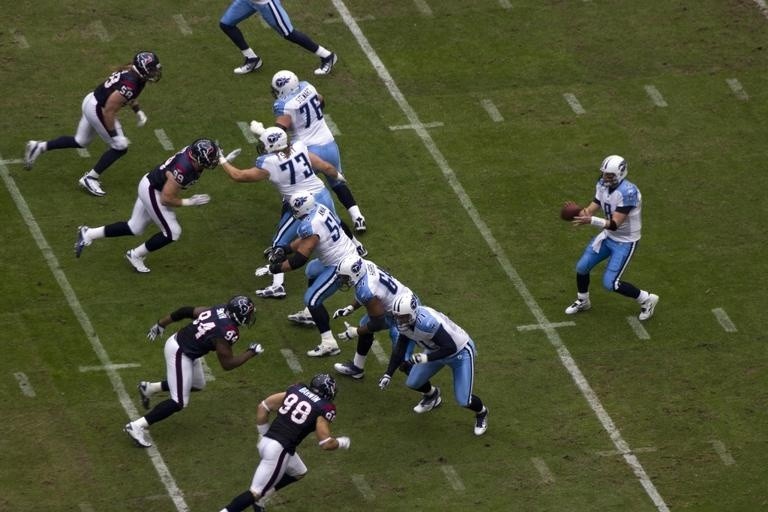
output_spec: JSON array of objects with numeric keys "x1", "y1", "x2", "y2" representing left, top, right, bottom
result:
[{"x1": 560, "y1": 204, "x2": 582, "y2": 222}]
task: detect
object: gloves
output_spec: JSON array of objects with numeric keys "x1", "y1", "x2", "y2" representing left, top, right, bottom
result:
[
  {"x1": 338, "y1": 321, "x2": 359, "y2": 342},
  {"x1": 378, "y1": 374, "x2": 391, "y2": 390},
  {"x1": 225, "y1": 148, "x2": 241, "y2": 164},
  {"x1": 246, "y1": 343, "x2": 264, "y2": 357},
  {"x1": 182, "y1": 193, "x2": 210, "y2": 207},
  {"x1": 267, "y1": 245, "x2": 286, "y2": 261},
  {"x1": 408, "y1": 353, "x2": 427, "y2": 365},
  {"x1": 217, "y1": 148, "x2": 226, "y2": 164},
  {"x1": 336, "y1": 436, "x2": 351, "y2": 450},
  {"x1": 333, "y1": 305, "x2": 354, "y2": 319},
  {"x1": 131, "y1": 104, "x2": 148, "y2": 127},
  {"x1": 146, "y1": 320, "x2": 165, "y2": 342},
  {"x1": 254, "y1": 263, "x2": 280, "y2": 277},
  {"x1": 250, "y1": 120, "x2": 265, "y2": 135},
  {"x1": 107, "y1": 129, "x2": 129, "y2": 148},
  {"x1": 336, "y1": 171, "x2": 347, "y2": 183}
]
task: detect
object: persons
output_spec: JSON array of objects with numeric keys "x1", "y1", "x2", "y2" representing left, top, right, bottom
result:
[
  {"x1": 254, "y1": 190, "x2": 357, "y2": 358},
  {"x1": 216, "y1": 127, "x2": 367, "y2": 300},
  {"x1": 73, "y1": 138, "x2": 241, "y2": 273},
  {"x1": 220, "y1": 0, "x2": 338, "y2": 77},
  {"x1": 332, "y1": 254, "x2": 427, "y2": 375},
  {"x1": 218, "y1": 371, "x2": 352, "y2": 512},
  {"x1": 377, "y1": 291, "x2": 490, "y2": 436},
  {"x1": 562, "y1": 154, "x2": 661, "y2": 323},
  {"x1": 122, "y1": 294, "x2": 266, "y2": 449},
  {"x1": 24, "y1": 50, "x2": 163, "y2": 196},
  {"x1": 250, "y1": 70, "x2": 369, "y2": 256}
]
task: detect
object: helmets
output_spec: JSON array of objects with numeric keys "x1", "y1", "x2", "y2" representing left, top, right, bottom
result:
[
  {"x1": 256, "y1": 127, "x2": 288, "y2": 155},
  {"x1": 189, "y1": 137, "x2": 218, "y2": 170},
  {"x1": 133, "y1": 50, "x2": 162, "y2": 84},
  {"x1": 310, "y1": 374, "x2": 336, "y2": 401},
  {"x1": 289, "y1": 190, "x2": 315, "y2": 219},
  {"x1": 335, "y1": 255, "x2": 366, "y2": 292},
  {"x1": 598, "y1": 155, "x2": 628, "y2": 187},
  {"x1": 227, "y1": 295, "x2": 257, "y2": 329},
  {"x1": 392, "y1": 294, "x2": 418, "y2": 332},
  {"x1": 269, "y1": 70, "x2": 299, "y2": 100}
]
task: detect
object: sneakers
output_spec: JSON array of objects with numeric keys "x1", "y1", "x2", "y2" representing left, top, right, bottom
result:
[
  {"x1": 73, "y1": 225, "x2": 89, "y2": 258},
  {"x1": 125, "y1": 248, "x2": 151, "y2": 273},
  {"x1": 234, "y1": 55, "x2": 263, "y2": 74},
  {"x1": 306, "y1": 341, "x2": 340, "y2": 357},
  {"x1": 638, "y1": 293, "x2": 659, "y2": 320},
  {"x1": 334, "y1": 361, "x2": 365, "y2": 380},
  {"x1": 564, "y1": 297, "x2": 592, "y2": 314},
  {"x1": 474, "y1": 407, "x2": 489, "y2": 435},
  {"x1": 255, "y1": 283, "x2": 286, "y2": 297},
  {"x1": 24, "y1": 140, "x2": 41, "y2": 171},
  {"x1": 413, "y1": 387, "x2": 442, "y2": 413},
  {"x1": 137, "y1": 381, "x2": 150, "y2": 410},
  {"x1": 355, "y1": 241, "x2": 369, "y2": 257},
  {"x1": 123, "y1": 421, "x2": 152, "y2": 448},
  {"x1": 78, "y1": 171, "x2": 106, "y2": 197},
  {"x1": 313, "y1": 52, "x2": 337, "y2": 75},
  {"x1": 252, "y1": 501, "x2": 265, "y2": 512},
  {"x1": 353, "y1": 216, "x2": 367, "y2": 231},
  {"x1": 288, "y1": 310, "x2": 316, "y2": 328}
]
